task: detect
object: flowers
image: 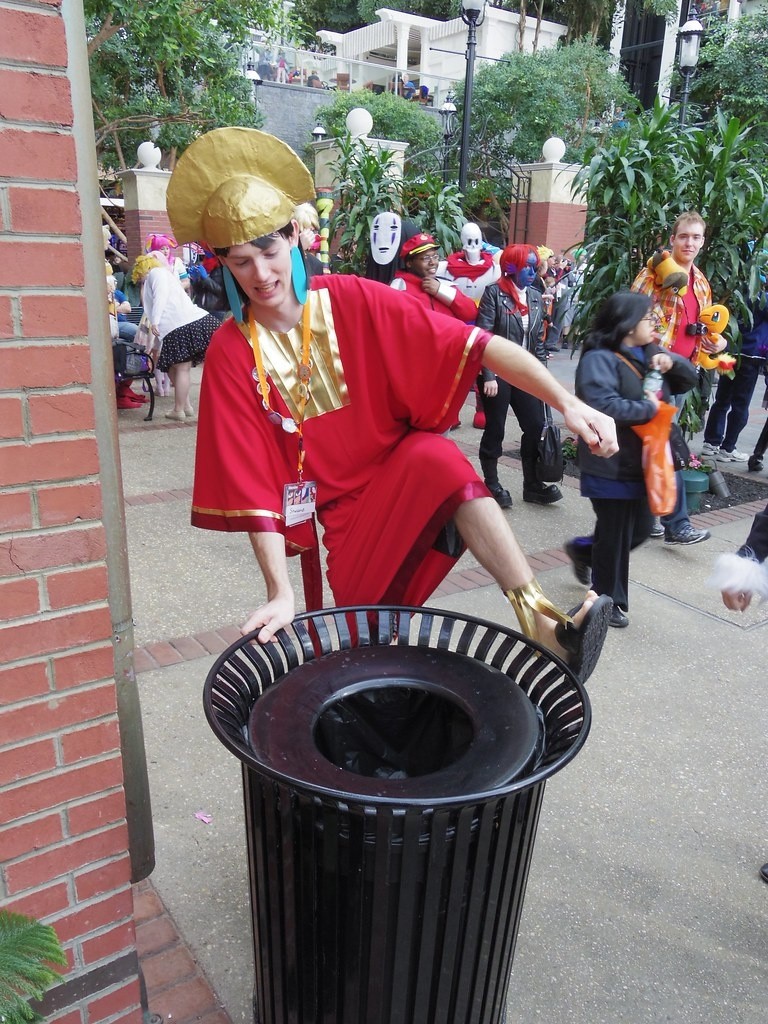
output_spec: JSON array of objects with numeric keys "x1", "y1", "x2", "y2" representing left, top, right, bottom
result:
[
  {"x1": 560, "y1": 439, "x2": 578, "y2": 458},
  {"x1": 686, "y1": 454, "x2": 712, "y2": 473}
]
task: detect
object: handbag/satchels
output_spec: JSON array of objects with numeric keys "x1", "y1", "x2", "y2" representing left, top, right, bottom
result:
[
  {"x1": 537, "y1": 426, "x2": 567, "y2": 482},
  {"x1": 630, "y1": 400, "x2": 678, "y2": 516}
]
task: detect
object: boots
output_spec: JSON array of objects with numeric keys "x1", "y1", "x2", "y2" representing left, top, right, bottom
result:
[
  {"x1": 562, "y1": 335, "x2": 569, "y2": 349},
  {"x1": 573, "y1": 334, "x2": 580, "y2": 350}
]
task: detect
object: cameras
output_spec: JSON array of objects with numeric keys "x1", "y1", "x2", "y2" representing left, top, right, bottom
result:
[{"x1": 686, "y1": 322, "x2": 709, "y2": 335}]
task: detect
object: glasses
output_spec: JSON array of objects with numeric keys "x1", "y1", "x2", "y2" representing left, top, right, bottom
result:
[
  {"x1": 642, "y1": 317, "x2": 657, "y2": 326},
  {"x1": 421, "y1": 255, "x2": 440, "y2": 262}
]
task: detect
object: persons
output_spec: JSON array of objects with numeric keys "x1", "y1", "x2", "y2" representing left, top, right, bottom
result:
[
  {"x1": 128, "y1": 254, "x2": 223, "y2": 420},
  {"x1": 533, "y1": 245, "x2": 597, "y2": 359},
  {"x1": 699, "y1": 277, "x2": 768, "y2": 473},
  {"x1": 561, "y1": 291, "x2": 661, "y2": 630},
  {"x1": 631, "y1": 209, "x2": 728, "y2": 547},
  {"x1": 293, "y1": 202, "x2": 325, "y2": 275},
  {"x1": 387, "y1": 232, "x2": 480, "y2": 326},
  {"x1": 476, "y1": 243, "x2": 563, "y2": 509},
  {"x1": 106, "y1": 274, "x2": 139, "y2": 342},
  {"x1": 707, "y1": 505, "x2": 768, "y2": 887},
  {"x1": 163, "y1": 124, "x2": 622, "y2": 693},
  {"x1": 136, "y1": 234, "x2": 230, "y2": 396}
]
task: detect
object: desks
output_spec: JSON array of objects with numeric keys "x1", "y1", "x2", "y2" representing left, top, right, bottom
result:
[{"x1": 329, "y1": 78, "x2": 356, "y2": 90}]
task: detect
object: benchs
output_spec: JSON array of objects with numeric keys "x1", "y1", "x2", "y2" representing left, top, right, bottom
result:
[{"x1": 112, "y1": 338, "x2": 154, "y2": 422}]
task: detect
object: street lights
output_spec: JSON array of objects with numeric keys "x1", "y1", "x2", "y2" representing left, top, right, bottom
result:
[
  {"x1": 676, "y1": 2, "x2": 706, "y2": 152},
  {"x1": 457, "y1": 0, "x2": 488, "y2": 215}
]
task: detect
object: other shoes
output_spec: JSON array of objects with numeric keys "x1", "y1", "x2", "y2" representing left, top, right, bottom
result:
[
  {"x1": 185, "y1": 406, "x2": 194, "y2": 416},
  {"x1": 546, "y1": 352, "x2": 550, "y2": 359},
  {"x1": 609, "y1": 605, "x2": 629, "y2": 627},
  {"x1": 164, "y1": 409, "x2": 185, "y2": 421},
  {"x1": 749, "y1": 457, "x2": 764, "y2": 471},
  {"x1": 549, "y1": 346, "x2": 561, "y2": 352},
  {"x1": 154, "y1": 389, "x2": 170, "y2": 397},
  {"x1": 565, "y1": 540, "x2": 590, "y2": 584}
]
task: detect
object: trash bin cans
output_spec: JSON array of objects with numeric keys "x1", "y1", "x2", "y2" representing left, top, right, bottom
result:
[{"x1": 202, "y1": 604, "x2": 590, "y2": 1024}]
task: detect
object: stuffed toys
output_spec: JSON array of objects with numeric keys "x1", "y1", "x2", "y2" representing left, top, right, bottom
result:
[
  {"x1": 692, "y1": 304, "x2": 736, "y2": 375},
  {"x1": 647, "y1": 247, "x2": 690, "y2": 297}
]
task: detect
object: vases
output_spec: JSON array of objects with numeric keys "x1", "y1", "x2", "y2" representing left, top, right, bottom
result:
[{"x1": 561, "y1": 437, "x2": 574, "y2": 446}]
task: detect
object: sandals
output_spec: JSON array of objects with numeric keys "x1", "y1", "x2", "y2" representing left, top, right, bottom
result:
[{"x1": 505, "y1": 580, "x2": 613, "y2": 683}]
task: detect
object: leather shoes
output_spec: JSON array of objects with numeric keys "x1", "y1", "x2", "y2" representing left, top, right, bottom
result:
[
  {"x1": 523, "y1": 481, "x2": 563, "y2": 504},
  {"x1": 491, "y1": 487, "x2": 513, "y2": 508}
]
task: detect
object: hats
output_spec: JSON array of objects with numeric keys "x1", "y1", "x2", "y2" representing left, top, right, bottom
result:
[
  {"x1": 166, "y1": 127, "x2": 317, "y2": 248},
  {"x1": 401, "y1": 234, "x2": 440, "y2": 260}
]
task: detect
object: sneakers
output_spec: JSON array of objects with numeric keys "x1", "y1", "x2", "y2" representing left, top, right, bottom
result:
[
  {"x1": 702, "y1": 443, "x2": 720, "y2": 455},
  {"x1": 718, "y1": 449, "x2": 749, "y2": 463},
  {"x1": 665, "y1": 525, "x2": 710, "y2": 545},
  {"x1": 650, "y1": 522, "x2": 666, "y2": 537}
]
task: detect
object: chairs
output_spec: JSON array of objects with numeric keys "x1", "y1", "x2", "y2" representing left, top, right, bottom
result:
[{"x1": 270, "y1": 64, "x2": 433, "y2": 107}]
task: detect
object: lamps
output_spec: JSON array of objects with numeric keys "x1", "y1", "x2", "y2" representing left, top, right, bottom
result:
[
  {"x1": 542, "y1": 137, "x2": 566, "y2": 163},
  {"x1": 137, "y1": 142, "x2": 162, "y2": 170},
  {"x1": 346, "y1": 108, "x2": 374, "y2": 138},
  {"x1": 312, "y1": 123, "x2": 327, "y2": 141}
]
